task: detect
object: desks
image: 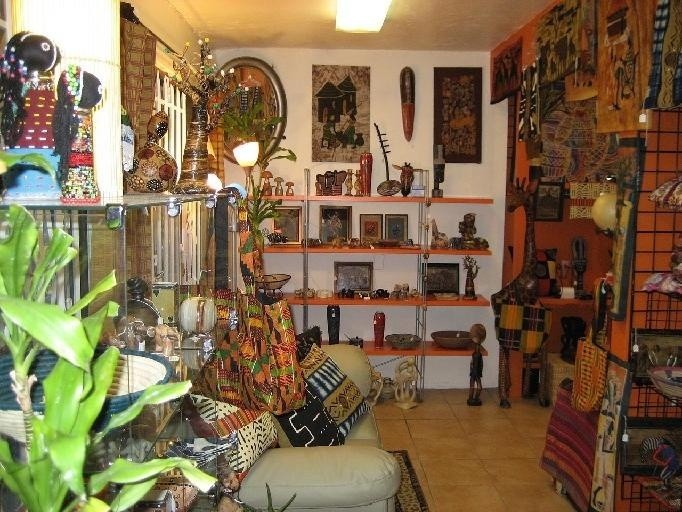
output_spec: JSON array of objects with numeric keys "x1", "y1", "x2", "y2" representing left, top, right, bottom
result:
[
  {"x1": 538, "y1": 298, "x2": 593, "y2": 408},
  {"x1": 538, "y1": 377, "x2": 600, "y2": 512}
]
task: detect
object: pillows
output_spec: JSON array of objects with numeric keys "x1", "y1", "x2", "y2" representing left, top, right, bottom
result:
[
  {"x1": 182, "y1": 392, "x2": 279, "y2": 491},
  {"x1": 649, "y1": 175, "x2": 682, "y2": 209},
  {"x1": 275, "y1": 342, "x2": 370, "y2": 446}
]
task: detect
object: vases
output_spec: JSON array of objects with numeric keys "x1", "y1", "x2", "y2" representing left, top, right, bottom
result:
[
  {"x1": 239, "y1": 296, "x2": 268, "y2": 417},
  {"x1": 211, "y1": 102, "x2": 297, "y2": 300}
]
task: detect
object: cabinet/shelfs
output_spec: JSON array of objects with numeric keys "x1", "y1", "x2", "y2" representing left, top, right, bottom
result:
[
  {"x1": 619, "y1": 111, "x2": 682, "y2": 511},
  {"x1": 246, "y1": 169, "x2": 494, "y2": 402}
]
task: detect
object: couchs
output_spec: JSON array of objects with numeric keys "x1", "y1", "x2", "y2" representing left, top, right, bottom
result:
[{"x1": 233, "y1": 345, "x2": 402, "y2": 511}]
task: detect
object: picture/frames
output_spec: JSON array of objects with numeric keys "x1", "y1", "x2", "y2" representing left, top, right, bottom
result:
[
  {"x1": 319, "y1": 205, "x2": 352, "y2": 244},
  {"x1": 271, "y1": 205, "x2": 302, "y2": 244},
  {"x1": 208, "y1": 56, "x2": 288, "y2": 168},
  {"x1": 334, "y1": 261, "x2": 373, "y2": 294},
  {"x1": 533, "y1": 182, "x2": 564, "y2": 223},
  {"x1": 433, "y1": 67, "x2": 483, "y2": 164},
  {"x1": 360, "y1": 214, "x2": 383, "y2": 244},
  {"x1": 385, "y1": 214, "x2": 408, "y2": 246},
  {"x1": 422, "y1": 263, "x2": 459, "y2": 295}
]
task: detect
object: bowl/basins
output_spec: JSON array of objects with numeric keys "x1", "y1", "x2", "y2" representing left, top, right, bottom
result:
[
  {"x1": 430, "y1": 331, "x2": 473, "y2": 349},
  {"x1": 649, "y1": 365, "x2": 682, "y2": 398},
  {"x1": 258, "y1": 274, "x2": 291, "y2": 290}
]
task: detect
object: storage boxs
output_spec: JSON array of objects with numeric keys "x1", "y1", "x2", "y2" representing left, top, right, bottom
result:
[{"x1": 542, "y1": 352, "x2": 575, "y2": 405}]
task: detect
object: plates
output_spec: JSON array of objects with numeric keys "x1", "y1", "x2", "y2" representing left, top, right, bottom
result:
[{"x1": 385, "y1": 334, "x2": 422, "y2": 349}]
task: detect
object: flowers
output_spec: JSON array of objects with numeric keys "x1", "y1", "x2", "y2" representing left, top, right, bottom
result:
[{"x1": 206, "y1": 143, "x2": 258, "y2": 298}]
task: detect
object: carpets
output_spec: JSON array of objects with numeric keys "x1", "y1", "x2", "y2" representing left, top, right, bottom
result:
[{"x1": 386, "y1": 449, "x2": 430, "y2": 512}]
